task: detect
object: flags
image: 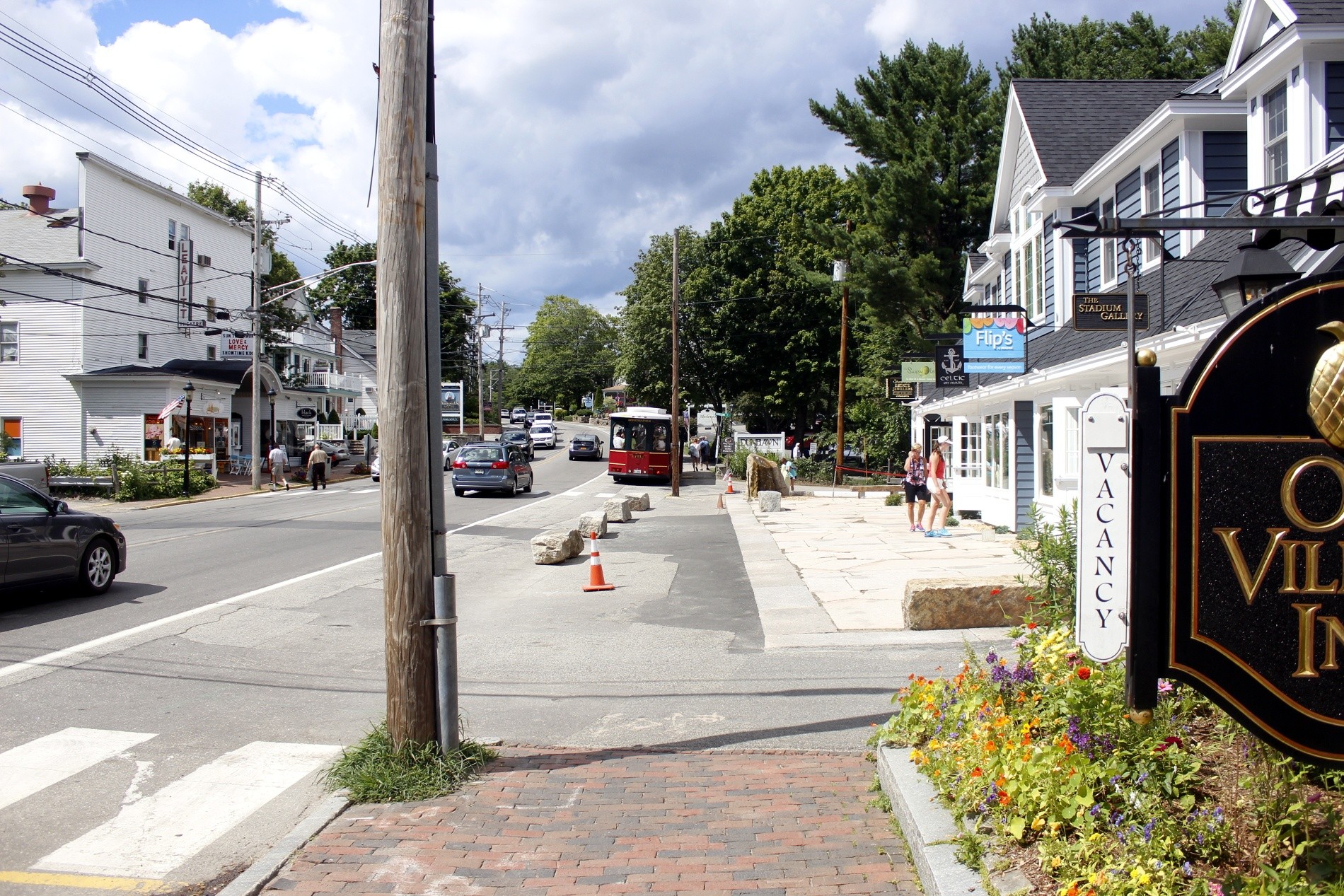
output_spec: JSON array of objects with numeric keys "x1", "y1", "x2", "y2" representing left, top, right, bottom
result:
[{"x1": 156, "y1": 394, "x2": 185, "y2": 420}]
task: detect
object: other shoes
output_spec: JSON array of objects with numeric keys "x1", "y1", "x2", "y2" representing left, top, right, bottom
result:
[
  {"x1": 699, "y1": 467, "x2": 710, "y2": 470},
  {"x1": 270, "y1": 488, "x2": 275, "y2": 491},
  {"x1": 286, "y1": 484, "x2": 289, "y2": 491},
  {"x1": 311, "y1": 484, "x2": 326, "y2": 490},
  {"x1": 693, "y1": 467, "x2": 698, "y2": 472}
]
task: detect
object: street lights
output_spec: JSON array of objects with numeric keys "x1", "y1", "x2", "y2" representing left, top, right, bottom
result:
[
  {"x1": 249, "y1": 260, "x2": 378, "y2": 491},
  {"x1": 267, "y1": 386, "x2": 278, "y2": 450},
  {"x1": 182, "y1": 380, "x2": 196, "y2": 496}
]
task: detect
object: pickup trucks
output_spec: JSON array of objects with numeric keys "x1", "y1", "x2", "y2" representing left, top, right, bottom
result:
[
  {"x1": 0, "y1": 461, "x2": 52, "y2": 503},
  {"x1": 510, "y1": 407, "x2": 527, "y2": 423}
]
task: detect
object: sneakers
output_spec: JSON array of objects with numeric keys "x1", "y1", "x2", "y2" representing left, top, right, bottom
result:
[
  {"x1": 910, "y1": 525, "x2": 915, "y2": 532},
  {"x1": 936, "y1": 528, "x2": 952, "y2": 536},
  {"x1": 924, "y1": 529, "x2": 940, "y2": 537},
  {"x1": 916, "y1": 524, "x2": 925, "y2": 532}
]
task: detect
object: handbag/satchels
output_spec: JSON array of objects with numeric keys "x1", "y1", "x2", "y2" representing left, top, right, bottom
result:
[
  {"x1": 700, "y1": 443, "x2": 706, "y2": 450},
  {"x1": 899, "y1": 478, "x2": 906, "y2": 489},
  {"x1": 687, "y1": 447, "x2": 695, "y2": 455}
]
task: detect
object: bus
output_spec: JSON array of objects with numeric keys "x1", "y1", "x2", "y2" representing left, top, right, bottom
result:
[{"x1": 608, "y1": 406, "x2": 684, "y2": 482}]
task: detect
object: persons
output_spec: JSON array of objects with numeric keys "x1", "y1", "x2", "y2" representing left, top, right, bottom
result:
[
  {"x1": 902, "y1": 443, "x2": 928, "y2": 532},
  {"x1": 793, "y1": 442, "x2": 801, "y2": 461},
  {"x1": 268, "y1": 442, "x2": 290, "y2": 492},
  {"x1": 166, "y1": 433, "x2": 181, "y2": 451},
  {"x1": 306, "y1": 443, "x2": 329, "y2": 490},
  {"x1": 687, "y1": 439, "x2": 701, "y2": 473},
  {"x1": 780, "y1": 458, "x2": 797, "y2": 492},
  {"x1": 923, "y1": 436, "x2": 953, "y2": 539},
  {"x1": 698, "y1": 435, "x2": 710, "y2": 471},
  {"x1": 613, "y1": 426, "x2": 672, "y2": 454}
]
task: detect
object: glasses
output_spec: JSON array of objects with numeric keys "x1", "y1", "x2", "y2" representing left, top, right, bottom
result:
[{"x1": 911, "y1": 450, "x2": 921, "y2": 454}]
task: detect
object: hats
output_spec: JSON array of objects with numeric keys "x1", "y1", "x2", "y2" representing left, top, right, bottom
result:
[
  {"x1": 938, "y1": 435, "x2": 954, "y2": 445},
  {"x1": 699, "y1": 436, "x2": 704, "y2": 439},
  {"x1": 657, "y1": 433, "x2": 666, "y2": 439}
]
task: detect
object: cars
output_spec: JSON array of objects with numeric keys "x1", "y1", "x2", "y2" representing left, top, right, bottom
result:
[
  {"x1": 300, "y1": 439, "x2": 351, "y2": 469},
  {"x1": 568, "y1": 434, "x2": 605, "y2": 461},
  {"x1": 0, "y1": 478, "x2": 128, "y2": 599},
  {"x1": 538, "y1": 423, "x2": 557, "y2": 440},
  {"x1": 371, "y1": 457, "x2": 379, "y2": 482},
  {"x1": 443, "y1": 440, "x2": 463, "y2": 470},
  {"x1": 451, "y1": 442, "x2": 534, "y2": 497},
  {"x1": 530, "y1": 425, "x2": 557, "y2": 449}
]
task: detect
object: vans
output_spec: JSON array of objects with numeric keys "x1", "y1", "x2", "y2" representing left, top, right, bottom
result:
[{"x1": 532, "y1": 413, "x2": 555, "y2": 426}]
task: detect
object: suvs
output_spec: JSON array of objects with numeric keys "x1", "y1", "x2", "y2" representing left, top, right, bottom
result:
[
  {"x1": 531, "y1": 409, "x2": 545, "y2": 413},
  {"x1": 495, "y1": 428, "x2": 534, "y2": 461},
  {"x1": 501, "y1": 409, "x2": 510, "y2": 418},
  {"x1": 524, "y1": 412, "x2": 540, "y2": 428}
]
